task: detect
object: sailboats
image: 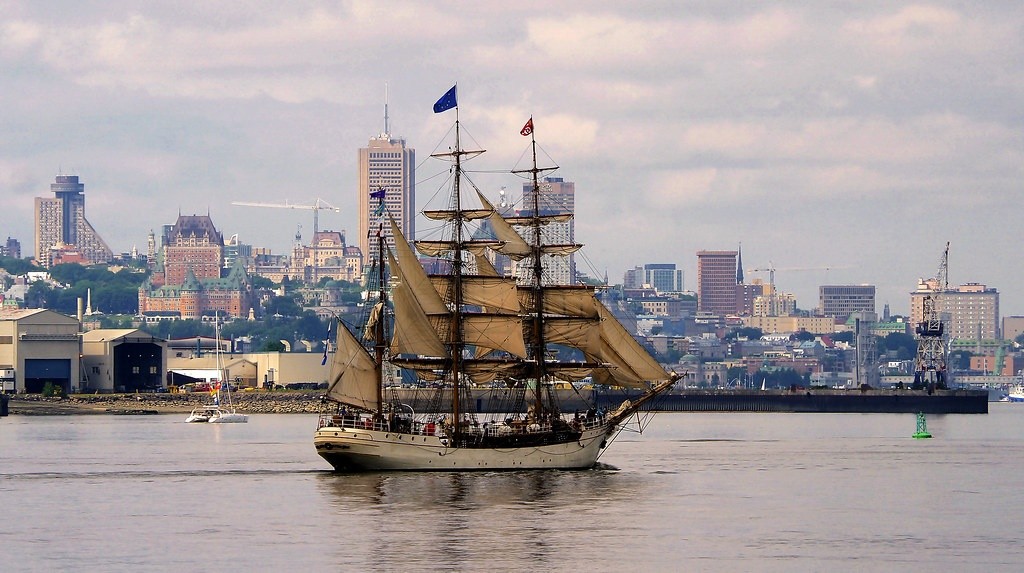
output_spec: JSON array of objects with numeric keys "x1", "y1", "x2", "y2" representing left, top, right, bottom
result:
[
  {"x1": 183, "y1": 311, "x2": 250, "y2": 423},
  {"x1": 312, "y1": 83, "x2": 689, "y2": 472}
]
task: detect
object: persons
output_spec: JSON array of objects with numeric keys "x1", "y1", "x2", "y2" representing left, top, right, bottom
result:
[{"x1": 336, "y1": 404, "x2": 607, "y2": 435}]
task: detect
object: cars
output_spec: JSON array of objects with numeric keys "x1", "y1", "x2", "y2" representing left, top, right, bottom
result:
[{"x1": 170, "y1": 371, "x2": 318, "y2": 391}]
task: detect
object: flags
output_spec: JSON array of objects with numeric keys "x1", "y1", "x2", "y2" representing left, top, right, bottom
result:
[
  {"x1": 520, "y1": 117, "x2": 532, "y2": 135},
  {"x1": 433, "y1": 85, "x2": 457, "y2": 112},
  {"x1": 369, "y1": 188, "x2": 384, "y2": 238},
  {"x1": 321, "y1": 321, "x2": 331, "y2": 365}
]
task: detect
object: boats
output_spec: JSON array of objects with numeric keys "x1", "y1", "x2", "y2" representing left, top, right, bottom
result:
[{"x1": 1001, "y1": 384, "x2": 1024, "y2": 402}]
